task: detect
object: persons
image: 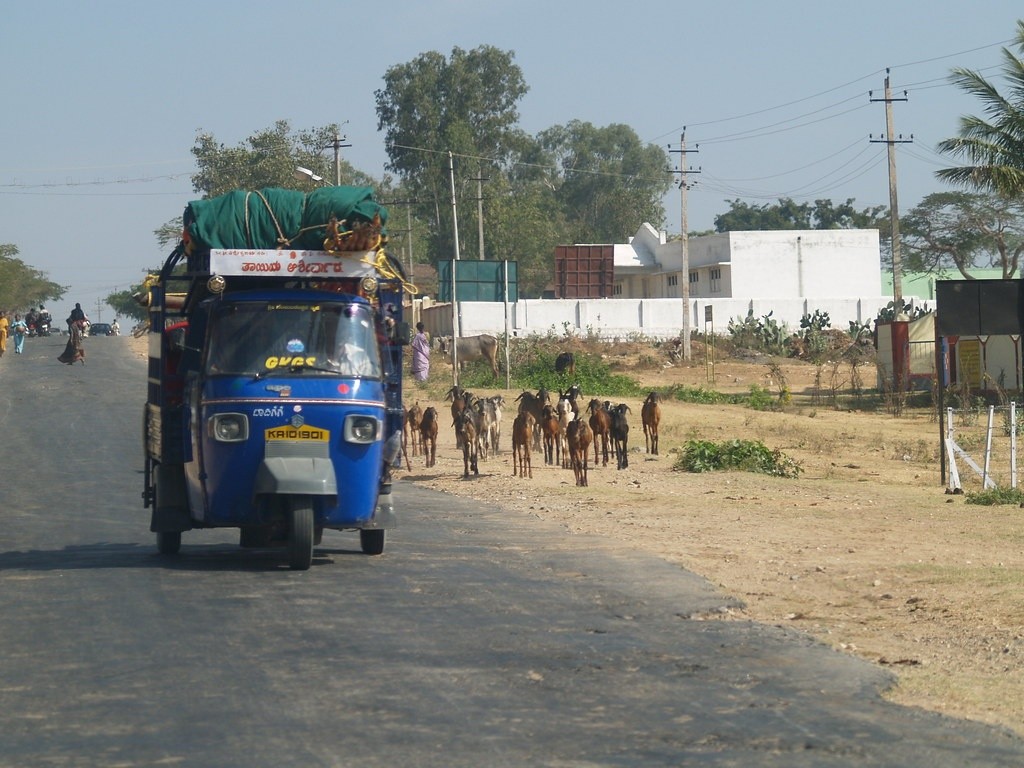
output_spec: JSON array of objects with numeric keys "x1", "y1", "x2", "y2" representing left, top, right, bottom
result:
[
  {"x1": 26, "y1": 305, "x2": 52, "y2": 337},
  {"x1": 11, "y1": 314, "x2": 30, "y2": 354},
  {"x1": 412, "y1": 322, "x2": 430, "y2": 380},
  {"x1": 67, "y1": 302, "x2": 91, "y2": 337},
  {"x1": 111, "y1": 319, "x2": 120, "y2": 335},
  {"x1": 313, "y1": 318, "x2": 373, "y2": 376},
  {"x1": 0, "y1": 312, "x2": 9, "y2": 357}
]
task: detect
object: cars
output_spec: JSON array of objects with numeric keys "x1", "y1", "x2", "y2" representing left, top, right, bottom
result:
[{"x1": 87, "y1": 323, "x2": 116, "y2": 337}]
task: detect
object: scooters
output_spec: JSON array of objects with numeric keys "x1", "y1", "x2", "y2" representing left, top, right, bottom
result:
[
  {"x1": 39, "y1": 319, "x2": 51, "y2": 336},
  {"x1": 28, "y1": 322, "x2": 40, "y2": 338}
]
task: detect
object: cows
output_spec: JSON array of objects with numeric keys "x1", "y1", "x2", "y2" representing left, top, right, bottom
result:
[{"x1": 437, "y1": 334, "x2": 500, "y2": 387}]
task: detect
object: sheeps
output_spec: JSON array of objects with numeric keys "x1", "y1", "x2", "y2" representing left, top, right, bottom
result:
[
  {"x1": 396, "y1": 385, "x2": 663, "y2": 487},
  {"x1": 556, "y1": 352, "x2": 575, "y2": 374}
]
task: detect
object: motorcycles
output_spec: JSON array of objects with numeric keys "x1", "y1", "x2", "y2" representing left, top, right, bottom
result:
[{"x1": 137, "y1": 241, "x2": 413, "y2": 568}]
